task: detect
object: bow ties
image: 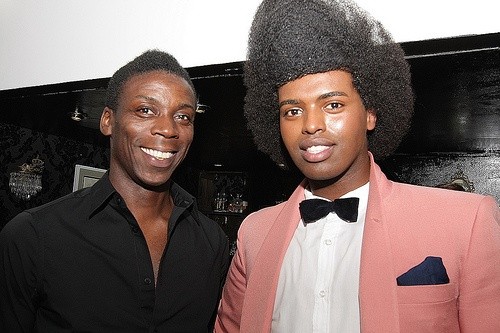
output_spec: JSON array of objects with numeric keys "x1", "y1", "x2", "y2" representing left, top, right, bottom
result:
[{"x1": 299, "y1": 197, "x2": 359, "y2": 227}]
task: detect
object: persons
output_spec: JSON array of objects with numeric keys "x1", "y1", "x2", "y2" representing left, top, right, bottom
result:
[
  {"x1": 212, "y1": 0, "x2": 500, "y2": 333},
  {"x1": 0, "y1": 51, "x2": 229, "y2": 333}
]
